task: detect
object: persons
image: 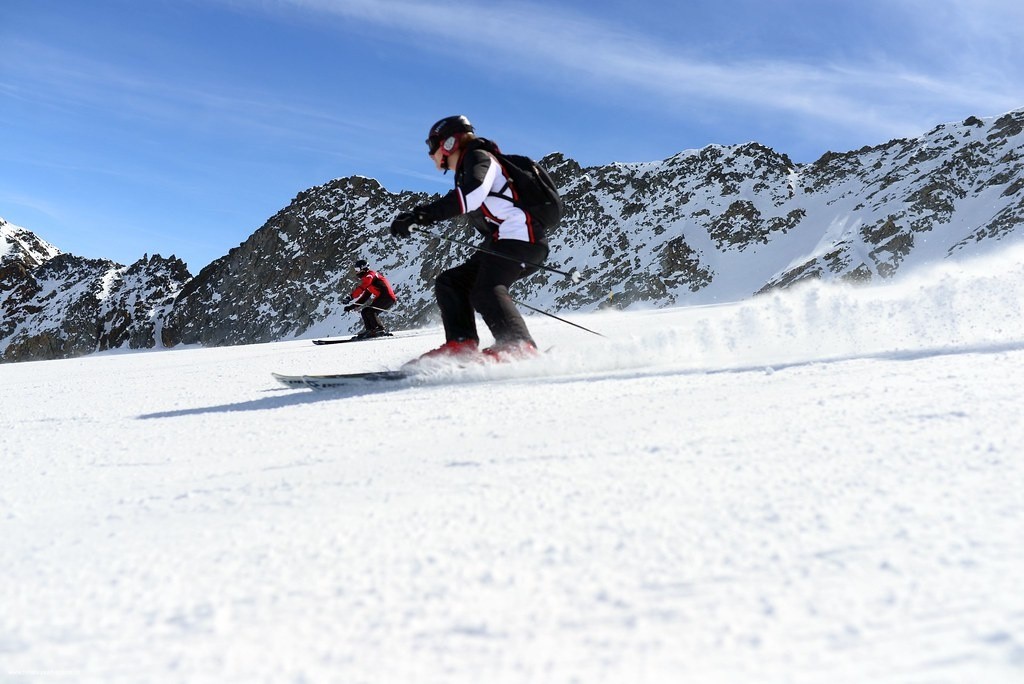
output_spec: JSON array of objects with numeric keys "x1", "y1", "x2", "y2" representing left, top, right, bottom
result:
[
  {"x1": 342, "y1": 260, "x2": 396, "y2": 339},
  {"x1": 389, "y1": 115, "x2": 564, "y2": 370}
]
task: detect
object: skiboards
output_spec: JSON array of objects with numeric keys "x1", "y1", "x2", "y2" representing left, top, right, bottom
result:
[
  {"x1": 312, "y1": 335, "x2": 396, "y2": 346},
  {"x1": 271, "y1": 346, "x2": 555, "y2": 387}
]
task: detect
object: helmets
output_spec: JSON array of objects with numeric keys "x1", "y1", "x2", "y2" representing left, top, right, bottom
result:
[
  {"x1": 354, "y1": 260, "x2": 370, "y2": 276},
  {"x1": 425, "y1": 115, "x2": 474, "y2": 150}
]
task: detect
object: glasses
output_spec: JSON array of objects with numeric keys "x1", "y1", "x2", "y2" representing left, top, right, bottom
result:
[{"x1": 425, "y1": 135, "x2": 440, "y2": 155}]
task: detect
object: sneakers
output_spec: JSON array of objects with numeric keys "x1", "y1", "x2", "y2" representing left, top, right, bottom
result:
[
  {"x1": 479, "y1": 340, "x2": 539, "y2": 364},
  {"x1": 420, "y1": 339, "x2": 479, "y2": 360},
  {"x1": 357, "y1": 326, "x2": 387, "y2": 338}
]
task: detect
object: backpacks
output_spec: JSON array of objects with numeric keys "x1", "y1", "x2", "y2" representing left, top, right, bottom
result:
[{"x1": 481, "y1": 147, "x2": 564, "y2": 241}]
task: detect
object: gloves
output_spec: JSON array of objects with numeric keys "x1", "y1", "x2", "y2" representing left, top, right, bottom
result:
[
  {"x1": 343, "y1": 296, "x2": 351, "y2": 304},
  {"x1": 344, "y1": 304, "x2": 355, "y2": 312},
  {"x1": 390, "y1": 203, "x2": 439, "y2": 238}
]
